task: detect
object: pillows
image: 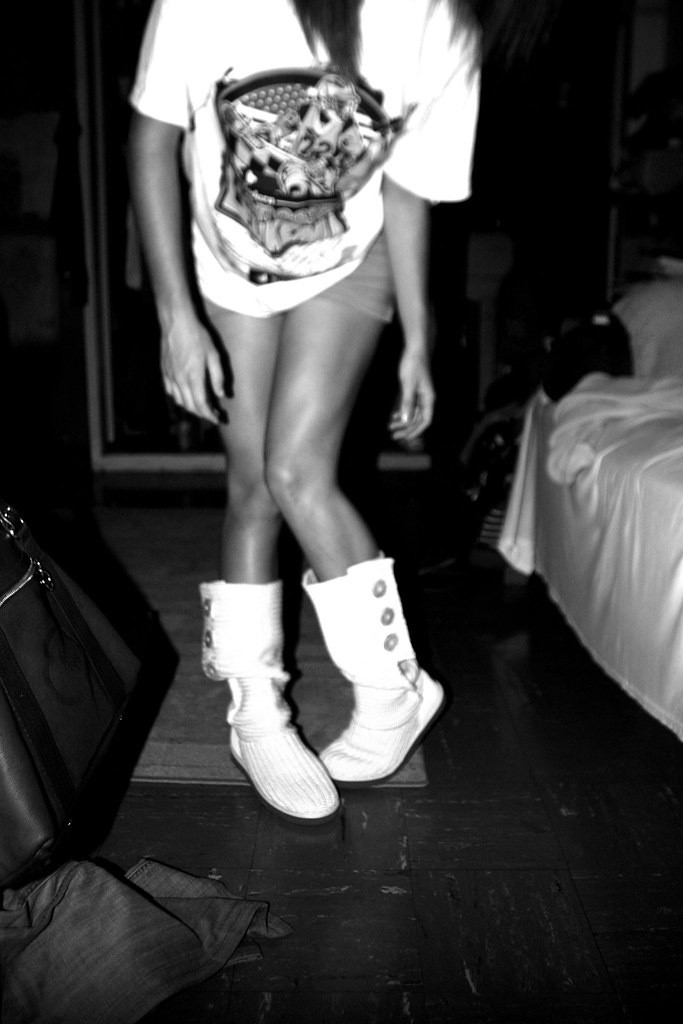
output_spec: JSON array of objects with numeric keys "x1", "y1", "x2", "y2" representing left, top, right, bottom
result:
[{"x1": 613, "y1": 279, "x2": 683, "y2": 378}]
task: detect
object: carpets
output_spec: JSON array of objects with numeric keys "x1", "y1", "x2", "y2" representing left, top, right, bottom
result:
[{"x1": 86, "y1": 505, "x2": 430, "y2": 789}]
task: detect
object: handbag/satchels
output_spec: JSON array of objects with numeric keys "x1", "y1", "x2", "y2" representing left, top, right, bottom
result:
[{"x1": 0, "y1": 495, "x2": 182, "y2": 885}]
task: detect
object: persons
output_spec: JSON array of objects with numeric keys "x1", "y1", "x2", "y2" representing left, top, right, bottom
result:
[{"x1": 124, "y1": 0, "x2": 497, "y2": 828}]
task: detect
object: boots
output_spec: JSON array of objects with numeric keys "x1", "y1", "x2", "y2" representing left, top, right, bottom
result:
[
  {"x1": 300, "y1": 549, "x2": 444, "y2": 790},
  {"x1": 200, "y1": 580, "x2": 341, "y2": 825}
]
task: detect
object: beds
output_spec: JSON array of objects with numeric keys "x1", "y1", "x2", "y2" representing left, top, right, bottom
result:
[{"x1": 495, "y1": 277, "x2": 683, "y2": 741}]
